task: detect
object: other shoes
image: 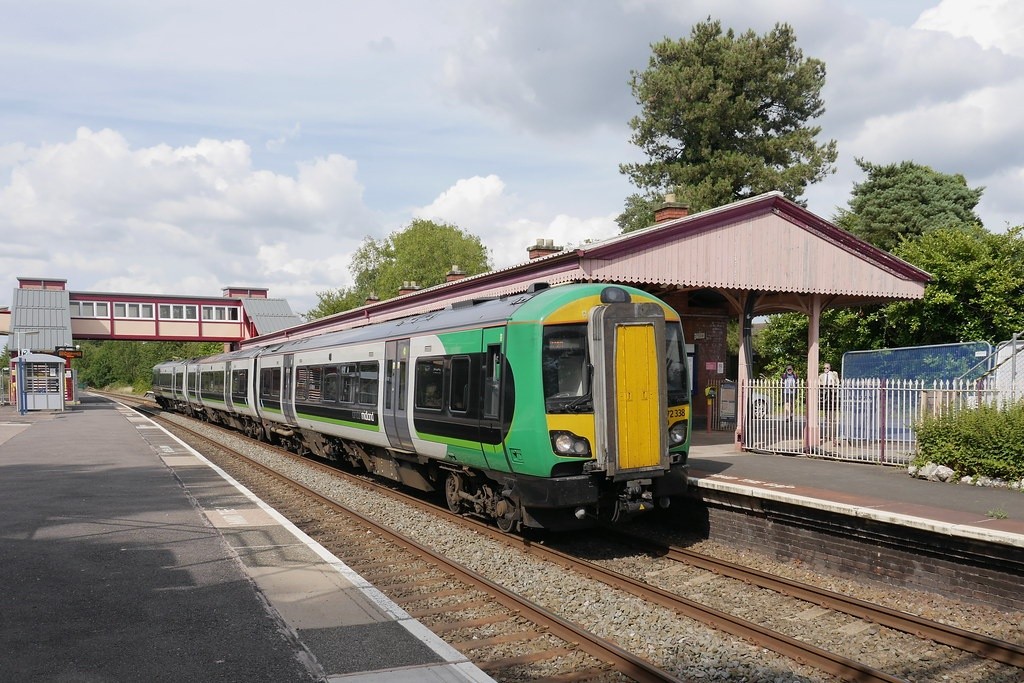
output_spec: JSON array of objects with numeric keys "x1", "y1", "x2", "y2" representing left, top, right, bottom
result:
[
  {"x1": 825, "y1": 418, "x2": 832, "y2": 422},
  {"x1": 786, "y1": 416, "x2": 793, "y2": 423}
]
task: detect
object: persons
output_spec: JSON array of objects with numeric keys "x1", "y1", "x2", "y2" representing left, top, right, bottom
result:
[
  {"x1": 818, "y1": 363, "x2": 840, "y2": 420},
  {"x1": 782, "y1": 363, "x2": 798, "y2": 420}
]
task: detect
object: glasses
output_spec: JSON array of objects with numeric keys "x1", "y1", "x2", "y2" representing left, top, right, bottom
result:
[{"x1": 823, "y1": 367, "x2": 829, "y2": 370}]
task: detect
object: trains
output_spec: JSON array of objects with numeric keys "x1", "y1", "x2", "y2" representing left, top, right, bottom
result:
[{"x1": 154, "y1": 280, "x2": 695, "y2": 541}]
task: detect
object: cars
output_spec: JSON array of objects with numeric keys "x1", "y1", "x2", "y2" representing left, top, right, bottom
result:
[{"x1": 721, "y1": 379, "x2": 773, "y2": 420}]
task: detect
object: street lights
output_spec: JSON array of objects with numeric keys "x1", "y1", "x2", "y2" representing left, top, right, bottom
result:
[{"x1": 17, "y1": 331, "x2": 40, "y2": 415}]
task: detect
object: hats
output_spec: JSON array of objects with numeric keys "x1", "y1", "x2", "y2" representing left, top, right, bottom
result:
[{"x1": 786, "y1": 365, "x2": 792, "y2": 370}]
